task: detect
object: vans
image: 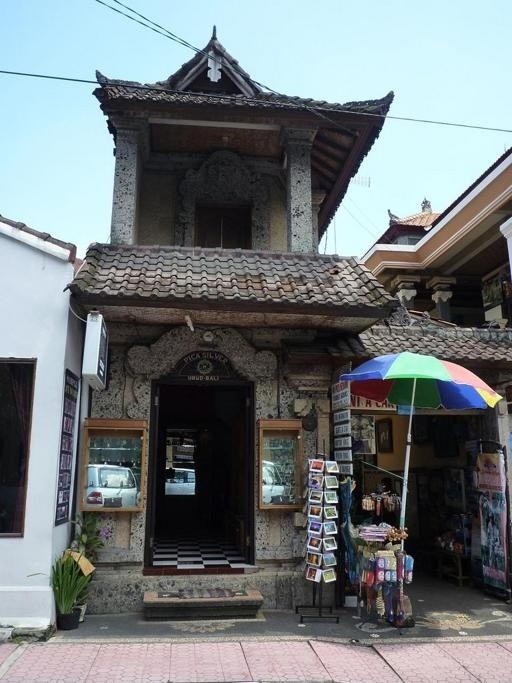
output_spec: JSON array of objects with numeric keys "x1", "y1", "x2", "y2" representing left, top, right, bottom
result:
[
  {"x1": 85, "y1": 461, "x2": 141, "y2": 509},
  {"x1": 261, "y1": 460, "x2": 286, "y2": 505},
  {"x1": 165, "y1": 466, "x2": 196, "y2": 496}
]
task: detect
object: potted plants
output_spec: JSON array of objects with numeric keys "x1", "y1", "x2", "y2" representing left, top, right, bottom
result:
[{"x1": 27, "y1": 552, "x2": 93, "y2": 629}]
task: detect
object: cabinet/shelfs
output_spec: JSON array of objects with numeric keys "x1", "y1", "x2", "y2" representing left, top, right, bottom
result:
[
  {"x1": 255, "y1": 418, "x2": 306, "y2": 510},
  {"x1": 82, "y1": 418, "x2": 148, "y2": 513}
]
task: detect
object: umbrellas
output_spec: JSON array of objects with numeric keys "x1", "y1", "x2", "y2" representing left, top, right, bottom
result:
[{"x1": 339, "y1": 351, "x2": 504, "y2": 592}]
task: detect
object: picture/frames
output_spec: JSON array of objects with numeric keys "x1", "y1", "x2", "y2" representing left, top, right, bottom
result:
[{"x1": 376, "y1": 418, "x2": 393, "y2": 453}]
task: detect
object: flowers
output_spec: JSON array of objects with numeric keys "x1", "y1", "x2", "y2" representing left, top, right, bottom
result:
[{"x1": 68, "y1": 514, "x2": 113, "y2": 562}]
